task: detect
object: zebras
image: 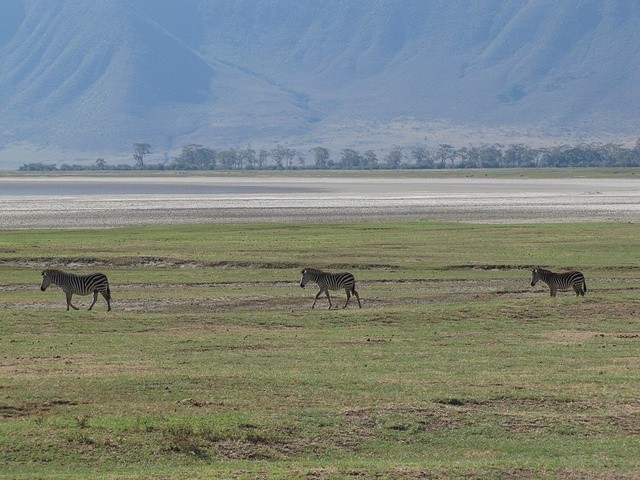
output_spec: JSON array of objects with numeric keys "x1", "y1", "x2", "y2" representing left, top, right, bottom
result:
[
  {"x1": 41, "y1": 269, "x2": 111, "y2": 311},
  {"x1": 529, "y1": 267, "x2": 587, "y2": 297},
  {"x1": 300, "y1": 268, "x2": 362, "y2": 310}
]
